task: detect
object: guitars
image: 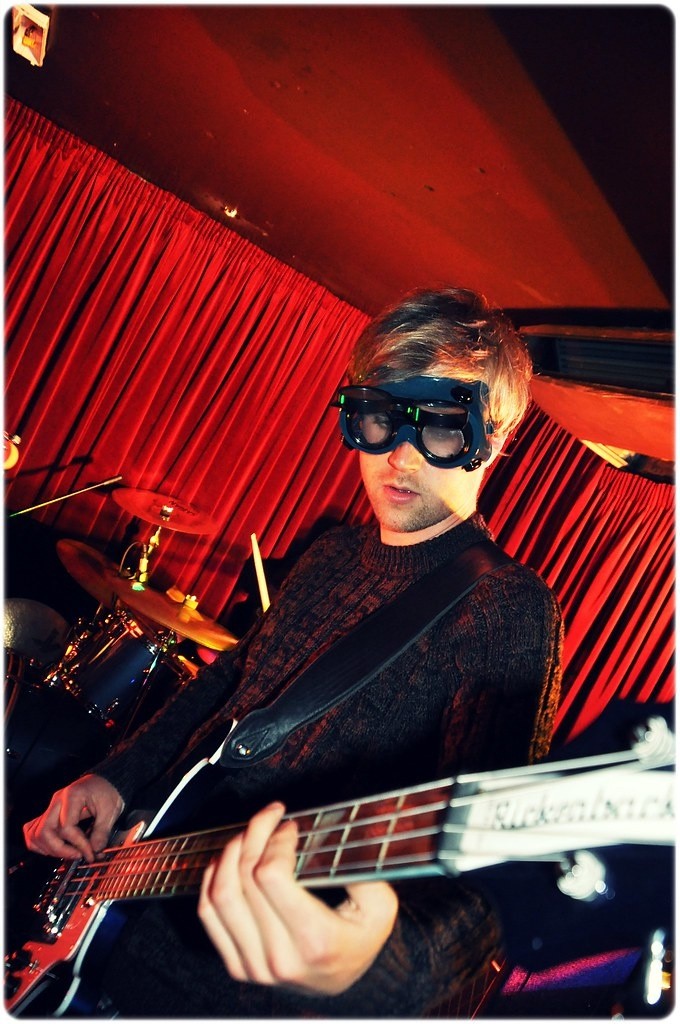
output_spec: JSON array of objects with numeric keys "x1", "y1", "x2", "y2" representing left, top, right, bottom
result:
[{"x1": 7, "y1": 710, "x2": 676, "y2": 1021}]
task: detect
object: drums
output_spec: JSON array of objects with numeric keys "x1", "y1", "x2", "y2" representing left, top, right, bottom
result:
[{"x1": 52, "y1": 610, "x2": 190, "y2": 741}]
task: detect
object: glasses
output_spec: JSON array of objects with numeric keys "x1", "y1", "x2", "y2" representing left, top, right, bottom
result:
[{"x1": 327, "y1": 374, "x2": 499, "y2": 474}]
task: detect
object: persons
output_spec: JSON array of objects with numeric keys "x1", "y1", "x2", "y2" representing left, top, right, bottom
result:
[{"x1": 21, "y1": 285, "x2": 564, "y2": 1017}]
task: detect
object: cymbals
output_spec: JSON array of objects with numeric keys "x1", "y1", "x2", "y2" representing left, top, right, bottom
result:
[
  {"x1": 113, "y1": 488, "x2": 216, "y2": 536},
  {"x1": 56, "y1": 537, "x2": 111, "y2": 606},
  {"x1": 111, "y1": 577, "x2": 239, "y2": 653}
]
